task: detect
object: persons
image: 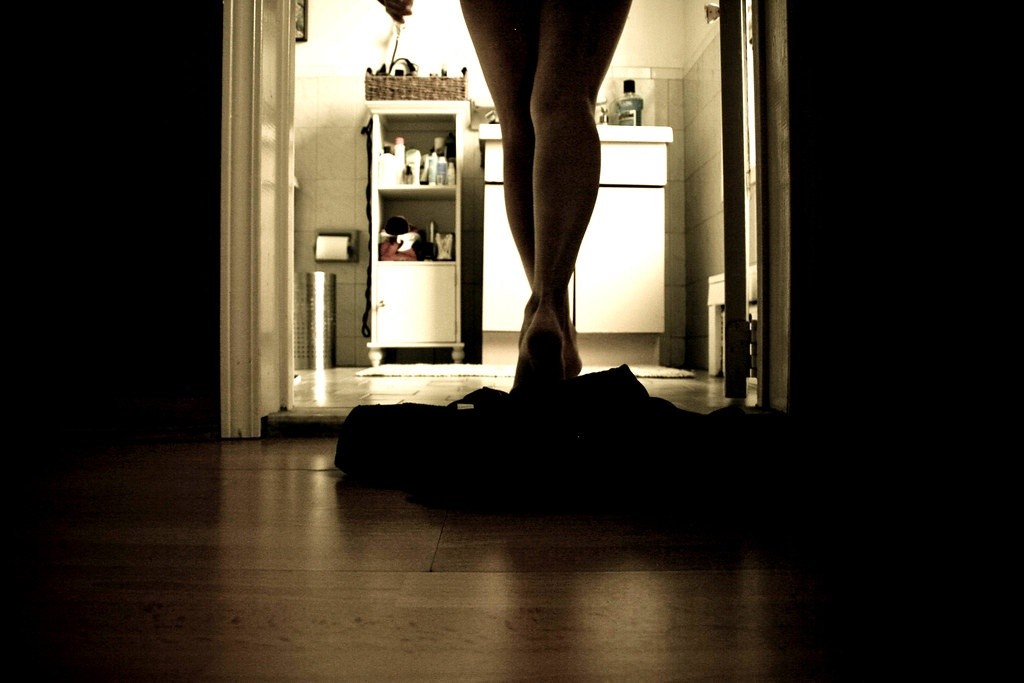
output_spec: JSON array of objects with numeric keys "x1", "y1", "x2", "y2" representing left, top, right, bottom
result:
[{"x1": 378, "y1": 0, "x2": 633, "y2": 393}]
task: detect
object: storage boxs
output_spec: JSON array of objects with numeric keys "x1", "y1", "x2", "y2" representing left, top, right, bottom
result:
[{"x1": 364, "y1": 65, "x2": 467, "y2": 100}]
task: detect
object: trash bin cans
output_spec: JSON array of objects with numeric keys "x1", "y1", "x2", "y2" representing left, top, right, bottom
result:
[{"x1": 293, "y1": 271, "x2": 337, "y2": 371}]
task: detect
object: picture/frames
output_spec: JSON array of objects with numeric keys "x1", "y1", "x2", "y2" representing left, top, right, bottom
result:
[{"x1": 294, "y1": 0, "x2": 307, "y2": 41}]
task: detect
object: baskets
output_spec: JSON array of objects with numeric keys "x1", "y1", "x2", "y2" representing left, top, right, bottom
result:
[{"x1": 365, "y1": 71, "x2": 468, "y2": 100}]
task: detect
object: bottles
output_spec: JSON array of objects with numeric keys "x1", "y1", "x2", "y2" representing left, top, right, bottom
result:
[
  {"x1": 436, "y1": 156, "x2": 448, "y2": 186},
  {"x1": 394, "y1": 136, "x2": 406, "y2": 174},
  {"x1": 616, "y1": 80, "x2": 643, "y2": 125},
  {"x1": 379, "y1": 146, "x2": 401, "y2": 189},
  {"x1": 445, "y1": 157, "x2": 455, "y2": 186}
]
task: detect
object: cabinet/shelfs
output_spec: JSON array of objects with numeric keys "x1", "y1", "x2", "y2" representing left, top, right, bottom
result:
[
  {"x1": 478, "y1": 122, "x2": 674, "y2": 367},
  {"x1": 361, "y1": 99, "x2": 470, "y2": 369}
]
